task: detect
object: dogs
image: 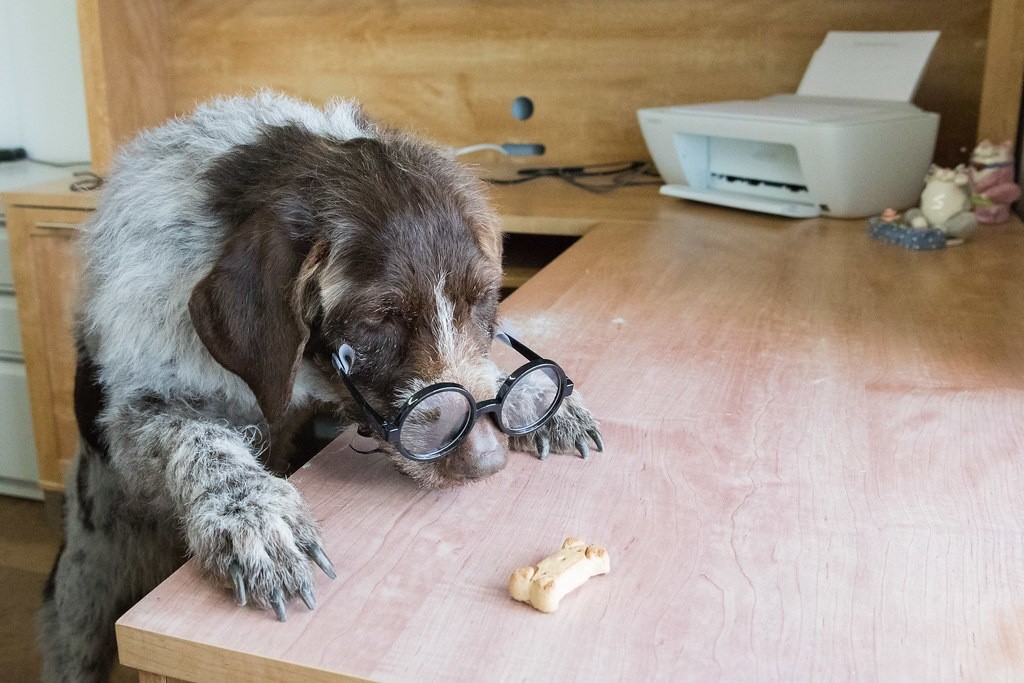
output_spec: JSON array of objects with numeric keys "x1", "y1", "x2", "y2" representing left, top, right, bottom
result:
[{"x1": 30, "y1": 86, "x2": 606, "y2": 683}]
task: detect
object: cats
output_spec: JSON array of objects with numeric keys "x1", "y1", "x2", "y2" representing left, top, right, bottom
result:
[
  {"x1": 956, "y1": 137, "x2": 1022, "y2": 227},
  {"x1": 903, "y1": 160, "x2": 982, "y2": 248}
]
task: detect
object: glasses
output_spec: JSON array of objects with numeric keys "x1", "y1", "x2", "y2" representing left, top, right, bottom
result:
[{"x1": 312, "y1": 323, "x2": 574, "y2": 463}]
